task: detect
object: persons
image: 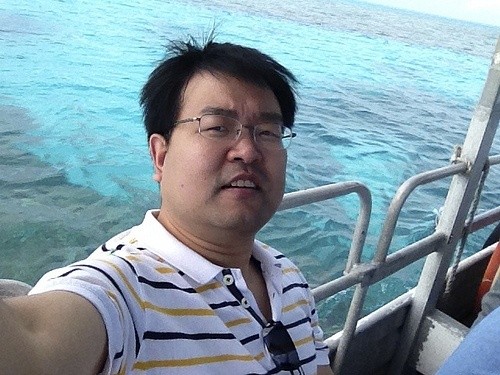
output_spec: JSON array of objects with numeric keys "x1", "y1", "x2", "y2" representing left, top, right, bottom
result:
[{"x1": 0, "y1": 15, "x2": 337, "y2": 375}]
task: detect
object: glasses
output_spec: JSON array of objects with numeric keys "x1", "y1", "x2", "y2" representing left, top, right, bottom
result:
[
  {"x1": 168, "y1": 113, "x2": 296, "y2": 150},
  {"x1": 262, "y1": 321, "x2": 307, "y2": 375}
]
